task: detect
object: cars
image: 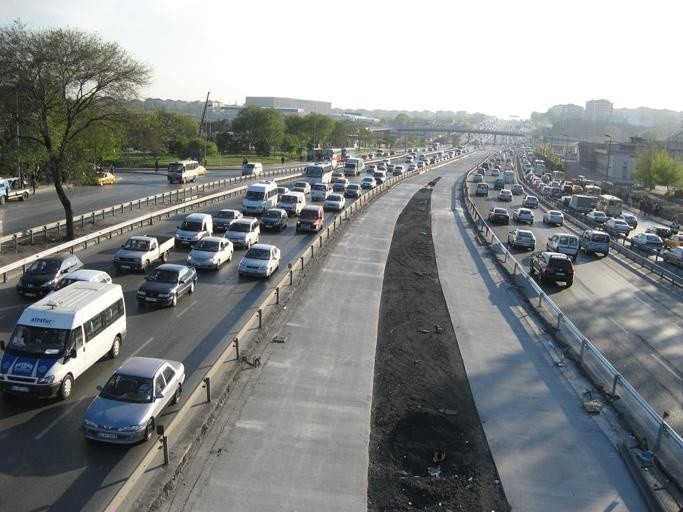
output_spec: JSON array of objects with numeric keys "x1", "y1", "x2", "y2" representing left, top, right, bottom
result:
[{"x1": 473, "y1": 134, "x2": 683, "y2": 286}]
